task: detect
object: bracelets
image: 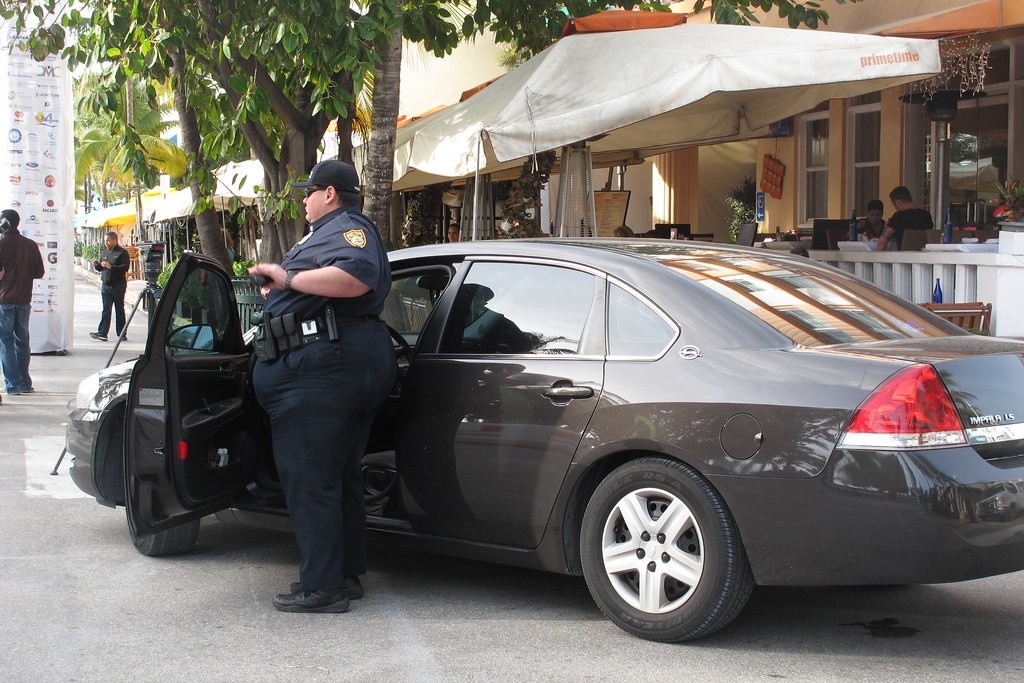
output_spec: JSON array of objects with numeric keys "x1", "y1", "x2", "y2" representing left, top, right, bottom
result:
[{"x1": 285, "y1": 271, "x2": 298, "y2": 291}]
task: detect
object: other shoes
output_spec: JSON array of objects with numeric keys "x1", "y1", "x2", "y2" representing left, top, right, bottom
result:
[
  {"x1": 1, "y1": 388, "x2": 20, "y2": 395},
  {"x1": 20, "y1": 386, "x2": 34, "y2": 392}
]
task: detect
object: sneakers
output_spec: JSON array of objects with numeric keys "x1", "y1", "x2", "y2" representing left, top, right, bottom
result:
[
  {"x1": 89, "y1": 332, "x2": 108, "y2": 340},
  {"x1": 119, "y1": 335, "x2": 128, "y2": 341}
]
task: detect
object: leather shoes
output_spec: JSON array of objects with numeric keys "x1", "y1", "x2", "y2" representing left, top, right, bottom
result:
[
  {"x1": 290, "y1": 576, "x2": 363, "y2": 600},
  {"x1": 272, "y1": 587, "x2": 350, "y2": 612}
]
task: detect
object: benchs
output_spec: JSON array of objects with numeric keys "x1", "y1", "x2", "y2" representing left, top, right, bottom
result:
[{"x1": 649, "y1": 217, "x2": 999, "y2": 252}]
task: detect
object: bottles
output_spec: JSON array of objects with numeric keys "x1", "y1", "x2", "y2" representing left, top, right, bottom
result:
[
  {"x1": 849, "y1": 209, "x2": 858, "y2": 241},
  {"x1": 946, "y1": 209, "x2": 952, "y2": 243},
  {"x1": 933, "y1": 278, "x2": 942, "y2": 303}
]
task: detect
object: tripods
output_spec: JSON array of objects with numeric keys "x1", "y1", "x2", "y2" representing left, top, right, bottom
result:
[{"x1": 50, "y1": 282, "x2": 168, "y2": 475}]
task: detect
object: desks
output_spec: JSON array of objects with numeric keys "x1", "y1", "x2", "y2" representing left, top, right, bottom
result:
[
  {"x1": 836, "y1": 237, "x2": 898, "y2": 252},
  {"x1": 765, "y1": 239, "x2": 811, "y2": 250},
  {"x1": 924, "y1": 242, "x2": 999, "y2": 253}
]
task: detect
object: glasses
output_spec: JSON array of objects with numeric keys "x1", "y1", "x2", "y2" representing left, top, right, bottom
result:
[{"x1": 305, "y1": 187, "x2": 326, "y2": 198}]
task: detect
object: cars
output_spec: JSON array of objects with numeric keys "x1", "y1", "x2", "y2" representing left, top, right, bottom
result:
[{"x1": 67, "y1": 236, "x2": 1024, "y2": 642}]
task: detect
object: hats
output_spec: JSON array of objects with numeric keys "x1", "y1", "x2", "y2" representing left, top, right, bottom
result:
[{"x1": 291, "y1": 158, "x2": 362, "y2": 193}]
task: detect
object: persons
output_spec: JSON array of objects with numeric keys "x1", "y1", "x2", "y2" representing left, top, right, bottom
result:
[
  {"x1": 0, "y1": 209, "x2": 45, "y2": 394},
  {"x1": 857, "y1": 186, "x2": 934, "y2": 251},
  {"x1": 790, "y1": 247, "x2": 809, "y2": 259},
  {"x1": 447, "y1": 223, "x2": 460, "y2": 243},
  {"x1": 404, "y1": 283, "x2": 540, "y2": 528},
  {"x1": 90, "y1": 231, "x2": 130, "y2": 340},
  {"x1": 245, "y1": 160, "x2": 398, "y2": 613},
  {"x1": 612, "y1": 227, "x2": 635, "y2": 237}
]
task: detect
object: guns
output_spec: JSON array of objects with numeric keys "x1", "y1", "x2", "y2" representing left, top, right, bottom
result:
[{"x1": 249, "y1": 310, "x2": 278, "y2": 361}]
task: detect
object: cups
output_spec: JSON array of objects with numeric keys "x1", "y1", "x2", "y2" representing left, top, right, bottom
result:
[{"x1": 961, "y1": 237, "x2": 979, "y2": 243}]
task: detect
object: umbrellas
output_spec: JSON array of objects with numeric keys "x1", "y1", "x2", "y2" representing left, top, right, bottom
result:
[
  {"x1": 315, "y1": 9, "x2": 941, "y2": 233},
  {"x1": 80, "y1": 158, "x2": 265, "y2": 254},
  {"x1": 948, "y1": 154, "x2": 1001, "y2": 193}
]
